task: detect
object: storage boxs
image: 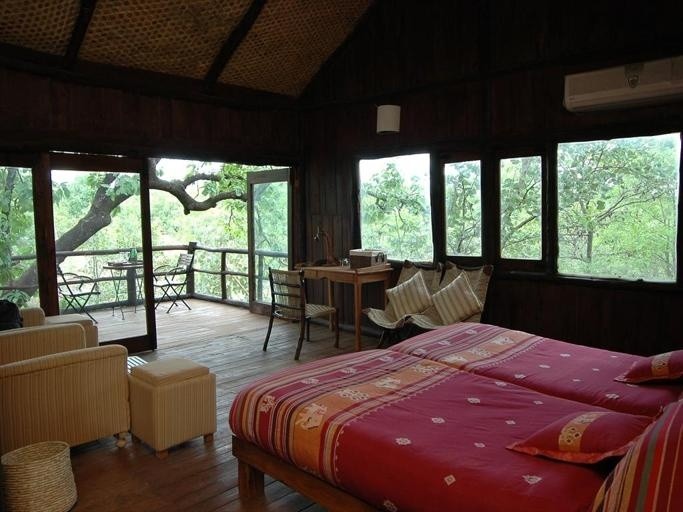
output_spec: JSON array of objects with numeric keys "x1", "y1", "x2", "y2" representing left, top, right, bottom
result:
[{"x1": 347, "y1": 248, "x2": 388, "y2": 270}]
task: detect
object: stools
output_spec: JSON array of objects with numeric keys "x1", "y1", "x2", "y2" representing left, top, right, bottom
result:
[
  {"x1": 44, "y1": 311, "x2": 100, "y2": 352},
  {"x1": 126, "y1": 357, "x2": 218, "y2": 460}
]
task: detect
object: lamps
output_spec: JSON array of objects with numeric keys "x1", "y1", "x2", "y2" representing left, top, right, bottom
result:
[
  {"x1": 374, "y1": 102, "x2": 403, "y2": 134},
  {"x1": 313, "y1": 225, "x2": 336, "y2": 265}
]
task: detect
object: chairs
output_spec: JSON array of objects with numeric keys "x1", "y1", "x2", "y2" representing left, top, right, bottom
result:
[
  {"x1": 55, "y1": 257, "x2": 102, "y2": 327},
  {"x1": 263, "y1": 264, "x2": 341, "y2": 362},
  {"x1": 0, "y1": 302, "x2": 77, "y2": 333},
  {"x1": 1, "y1": 322, "x2": 133, "y2": 458},
  {"x1": 412, "y1": 259, "x2": 497, "y2": 330},
  {"x1": 362, "y1": 256, "x2": 444, "y2": 348},
  {"x1": 152, "y1": 252, "x2": 196, "y2": 317}
]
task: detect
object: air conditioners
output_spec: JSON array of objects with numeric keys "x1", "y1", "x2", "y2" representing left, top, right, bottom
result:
[{"x1": 559, "y1": 56, "x2": 682, "y2": 112}]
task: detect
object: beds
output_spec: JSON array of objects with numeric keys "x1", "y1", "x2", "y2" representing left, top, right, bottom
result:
[{"x1": 224, "y1": 320, "x2": 681, "y2": 512}]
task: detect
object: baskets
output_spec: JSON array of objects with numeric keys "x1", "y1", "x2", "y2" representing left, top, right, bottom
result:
[{"x1": 1, "y1": 441, "x2": 78, "y2": 512}]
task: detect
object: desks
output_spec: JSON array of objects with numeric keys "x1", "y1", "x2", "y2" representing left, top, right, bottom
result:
[
  {"x1": 102, "y1": 260, "x2": 148, "y2": 322},
  {"x1": 293, "y1": 261, "x2": 393, "y2": 353}
]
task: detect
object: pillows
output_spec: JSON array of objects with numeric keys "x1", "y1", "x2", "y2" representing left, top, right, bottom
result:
[
  {"x1": 385, "y1": 268, "x2": 432, "y2": 319},
  {"x1": 504, "y1": 408, "x2": 657, "y2": 466},
  {"x1": 610, "y1": 345, "x2": 681, "y2": 388},
  {"x1": 429, "y1": 269, "x2": 483, "y2": 326}
]
